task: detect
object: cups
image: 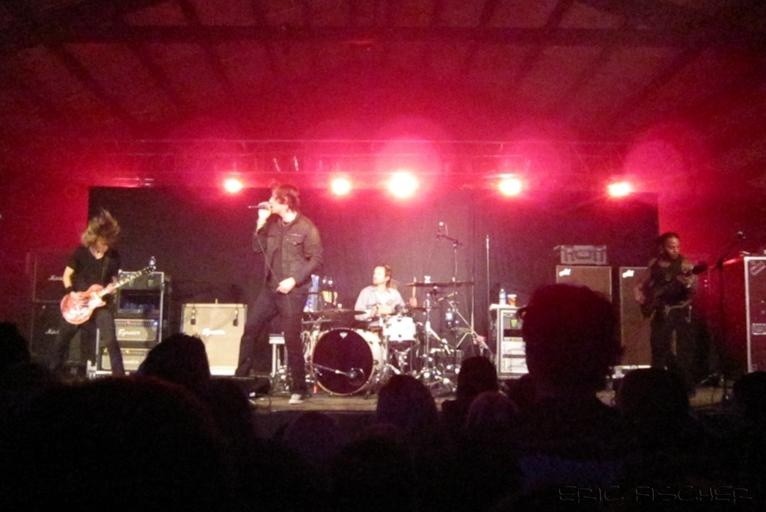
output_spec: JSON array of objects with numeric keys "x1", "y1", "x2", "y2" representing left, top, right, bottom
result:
[
  {"x1": 507, "y1": 294, "x2": 517, "y2": 306},
  {"x1": 510, "y1": 320, "x2": 518, "y2": 328}
]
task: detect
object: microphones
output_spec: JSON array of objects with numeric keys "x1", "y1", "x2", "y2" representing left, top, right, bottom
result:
[
  {"x1": 436, "y1": 220, "x2": 446, "y2": 240},
  {"x1": 247, "y1": 202, "x2": 273, "y2": 210}
]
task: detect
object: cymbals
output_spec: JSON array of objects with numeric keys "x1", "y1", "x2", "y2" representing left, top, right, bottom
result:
[
  {"x1": 406, "y1": 279, "x2": 466, "y2": 288},
  {"x1": 312, "y1": 310, "x2": 364, "y2": 317}
]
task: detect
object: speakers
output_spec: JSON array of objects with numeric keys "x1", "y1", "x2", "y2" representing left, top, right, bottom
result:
[
  {"x1": 549, "y1": 263, "x2": 617, "y2": 367},
  {"x1": 32, "y1": 248, "x2": 77, "y2": 301},
  {"x1": 614, "y1": 264, "x2": 657, "y2": 367},
  {"x1": 28, "y1": 304, "x2": 91, "y2": 368}
]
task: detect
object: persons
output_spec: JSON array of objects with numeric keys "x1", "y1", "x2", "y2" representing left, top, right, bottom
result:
[
  {"x1": 634, "y1": 232, "x2": 696, "y2": 369},
  {"x1": 242, "y1": 180, "x2": 323, "y2": 404},
  {"x1": 48, "y1": 208, "x2": 128, "y2": 376},
  {"x1": 353, "y1": 264, "x2": 418, "y2": 371}
]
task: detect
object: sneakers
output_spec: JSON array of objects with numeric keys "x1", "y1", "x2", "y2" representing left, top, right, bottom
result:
[{"x1": 287, "y1": 392, "x2": 306, "y2": 404}]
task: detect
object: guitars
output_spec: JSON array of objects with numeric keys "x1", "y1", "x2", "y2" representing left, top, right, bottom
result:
[
  {"x1": 61, "y1": 265, "x2": 155, "y2": 326},
  {"x1": 638, "y1": 260, "x2": 708, "y2": 317}
]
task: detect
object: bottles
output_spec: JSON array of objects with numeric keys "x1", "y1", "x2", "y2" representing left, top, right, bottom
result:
[
  {"x1": 148, "y1": 256, "x2": 157, "y2": 270},
  {"x1": 499, "y1": 288, "x2": 506, "y2": 305}
]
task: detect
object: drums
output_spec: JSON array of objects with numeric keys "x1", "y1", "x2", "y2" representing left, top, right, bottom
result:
[
  {"x1": 311, "y1": 328, "x2": 383, "y2": 397},
  {"x1": 385, "y1": 314, "x2": 417, "y2": 346}
]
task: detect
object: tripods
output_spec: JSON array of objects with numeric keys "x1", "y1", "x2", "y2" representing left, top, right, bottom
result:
[{"x1": 366, "y1": 244, "x2": 486, "y2": 399}]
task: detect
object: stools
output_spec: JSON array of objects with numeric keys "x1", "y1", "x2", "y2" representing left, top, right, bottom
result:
[{"x1": 267, "y1": 332, "x2": 288, "y2": 377}]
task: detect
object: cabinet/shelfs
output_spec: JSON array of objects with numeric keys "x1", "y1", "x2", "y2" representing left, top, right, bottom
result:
[
  {"x1": 93, "y1": 268, "x2": 172, "y2": 377},
  {"x1": 486, "y1": 305, "x2": 530, "y2": 383}
]
